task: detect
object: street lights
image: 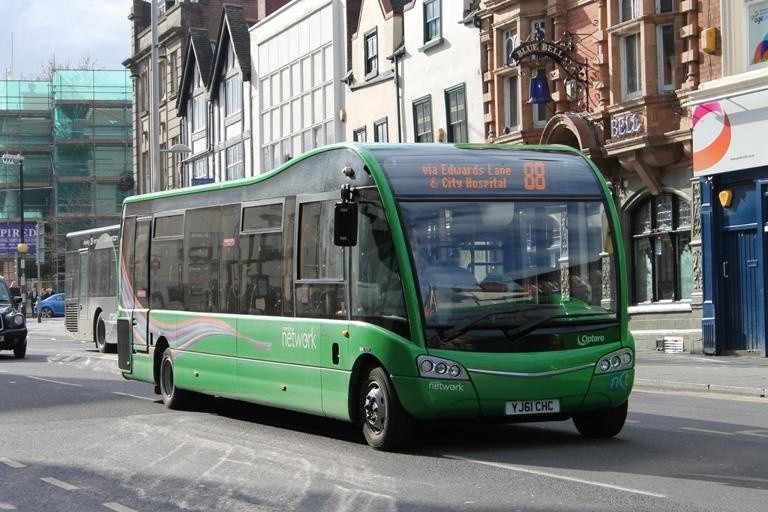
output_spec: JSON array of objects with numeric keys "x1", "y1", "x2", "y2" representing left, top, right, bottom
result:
[{"x1": 2, "y1": 154, "x2": 40, "y2": 320}]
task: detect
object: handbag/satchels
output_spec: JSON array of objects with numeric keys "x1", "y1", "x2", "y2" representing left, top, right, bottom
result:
[{"x1": 13, "y1": 296, "x2": 22, "y2": 303}]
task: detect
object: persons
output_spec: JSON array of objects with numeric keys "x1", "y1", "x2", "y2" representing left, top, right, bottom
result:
[
  {"x1": 8, "y1": 280, "x2": 21, "y2": 296},
  {"x1": 35, "y1": 296, "x2": 42, "y2": 323},
  {"x1": 29, "y1": 287, "x2": 56, "y2": 317}
]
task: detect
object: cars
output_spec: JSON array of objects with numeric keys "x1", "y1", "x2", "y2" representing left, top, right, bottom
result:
[
  {"x1": 0, "y1": 274, "x2": 28, "y2": 359},
  {"x1": 33, "y1": 291, "x2": 64, "y2": 318}
]
